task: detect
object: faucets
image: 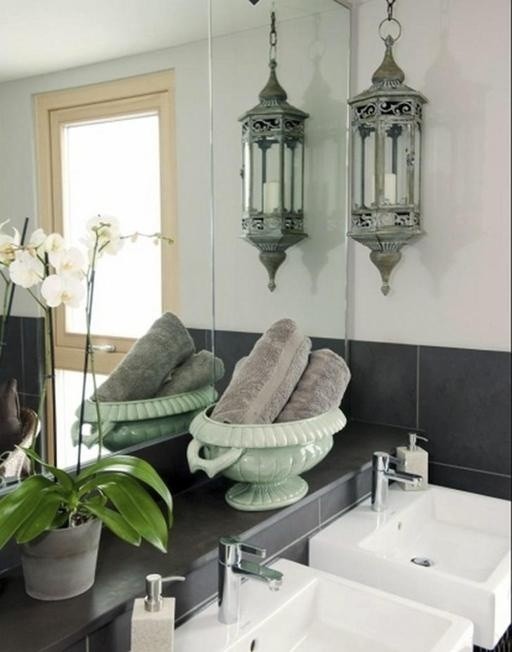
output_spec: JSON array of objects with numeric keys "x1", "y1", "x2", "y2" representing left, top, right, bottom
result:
[
  {"x1": 216, "y1": 536, "x2": 285, "y2": 625},
  {"x1": 369, "y1": 451, "x2": 424, "y2": 512}
]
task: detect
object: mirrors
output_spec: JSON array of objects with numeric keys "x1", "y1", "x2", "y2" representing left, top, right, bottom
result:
[{"x1": 0, "y1": 0, "x2": 360, "y2": 500}]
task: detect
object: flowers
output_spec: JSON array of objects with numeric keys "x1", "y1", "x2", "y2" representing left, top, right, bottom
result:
[
  {"x1": 0, "y1": 214, "x2": 175, "y2": 555},
  {"x1": 0, "y1": 217, "x2": 58, "y2": 485}
]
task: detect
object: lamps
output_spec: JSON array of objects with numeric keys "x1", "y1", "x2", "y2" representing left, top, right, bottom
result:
[
  {"x1": 237, "y1": 0, "x2": 311, "y2": 294},
  {"x1": 346, "y1": 1, "x2": 429, "y2": 297}
]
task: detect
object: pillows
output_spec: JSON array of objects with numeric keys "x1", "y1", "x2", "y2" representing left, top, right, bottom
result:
[{"x1": 0, "y1": 376, "x2": 23, "y2": 457}]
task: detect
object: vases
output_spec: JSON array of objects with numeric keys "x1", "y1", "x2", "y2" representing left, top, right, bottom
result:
[{"x1": 19, "y1": 514, "x2": 104, "y2": 601}]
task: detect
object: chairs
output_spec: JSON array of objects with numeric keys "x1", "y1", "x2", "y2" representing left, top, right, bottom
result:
[{"x1": 0, "y1": 405, "x2": 41, "y2": 488}]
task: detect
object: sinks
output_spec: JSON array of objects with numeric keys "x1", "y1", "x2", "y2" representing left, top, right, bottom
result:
[
  {"x1": 168, "y1": 557, "x2": 476, "y2": 651},
  {"x1": 307, "y1": 478, "x2": 511, "y2": 651}
]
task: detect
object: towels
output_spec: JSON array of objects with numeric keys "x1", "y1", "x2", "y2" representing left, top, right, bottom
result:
[
  {"x1": 88, "y1": 311, "x2": 226, "y2": 401},
  {"x1": 211, "y1": 317, "x2": 353, "y2": 425}
]
